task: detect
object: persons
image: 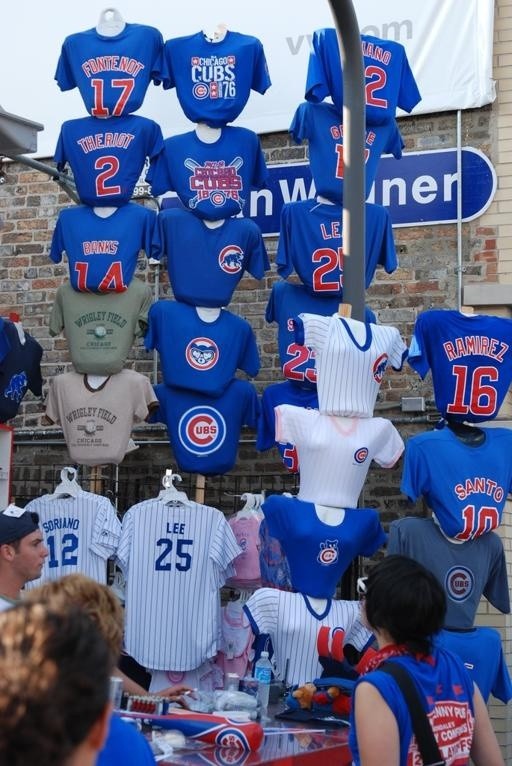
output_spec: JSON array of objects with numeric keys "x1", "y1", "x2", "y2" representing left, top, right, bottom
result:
[
  {"x1": 349, "y1": 554, "x2": 506, "y2": 766},
  {"x1": 0, "y1": 600, "x2": 114, "y2": 766},
  {"x1": 26, "y1": 572, "x2": 157, "y2": 766},
  {"x1": 0, "y1": 503, "x2": 199, "y2": 709}
]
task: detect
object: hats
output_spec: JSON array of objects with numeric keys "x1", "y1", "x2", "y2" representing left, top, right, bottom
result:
[{"x1": 0, "y1": 504, "x2": 41, "y2": 546}]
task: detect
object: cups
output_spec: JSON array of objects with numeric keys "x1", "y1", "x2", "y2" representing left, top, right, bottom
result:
[{"x1": 239, "y1": 679, "x2": 259, "y2": 698}]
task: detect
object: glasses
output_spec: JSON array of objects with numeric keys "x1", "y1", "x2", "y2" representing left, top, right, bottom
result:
[{"x1": 356, "y1": 577, "x2": 369, "y2": 595}]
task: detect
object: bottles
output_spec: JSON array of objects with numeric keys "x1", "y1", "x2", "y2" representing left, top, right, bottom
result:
[{"x1": 255, "y1": 651, "x2": 271, "y2": 708}]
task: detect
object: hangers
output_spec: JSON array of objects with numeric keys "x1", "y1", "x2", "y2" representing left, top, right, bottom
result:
[
  {"x1": 155, "y1": 474, "x2": 189, "y2": 508},
  {"x1": 50, "y1": 467, "x2": 83, "y2": 501},
  {"x1": 235, "y1": 492, "x2": 266, "y2": 523}
]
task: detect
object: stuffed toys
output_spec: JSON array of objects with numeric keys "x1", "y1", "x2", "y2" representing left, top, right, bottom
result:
[{"x1": 285, "y1": 683, "x2": 351, "y2": 714}]
